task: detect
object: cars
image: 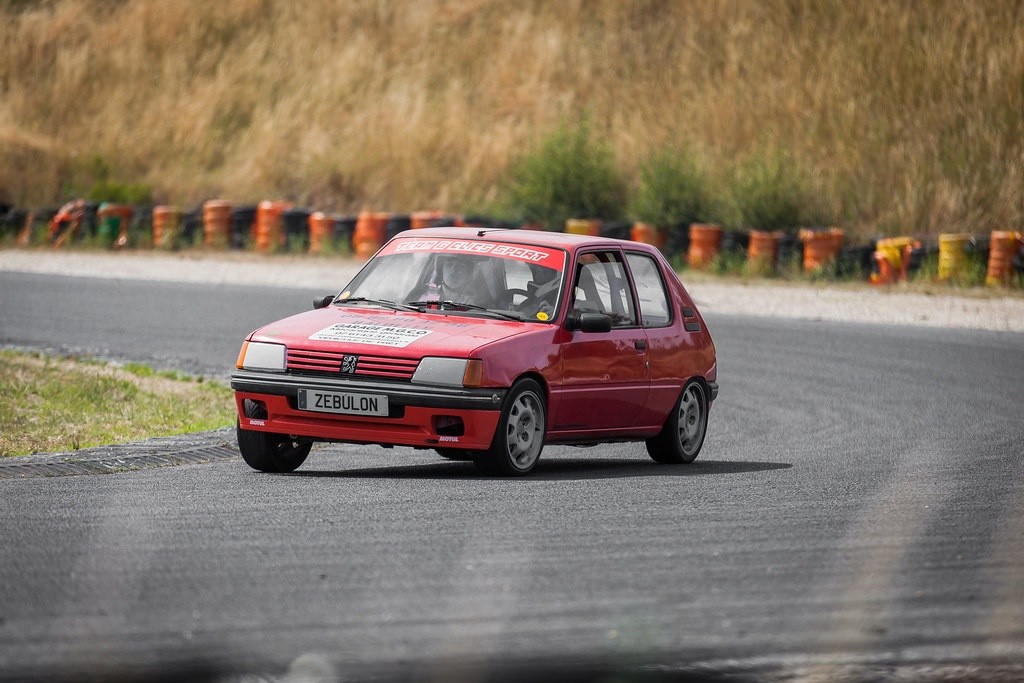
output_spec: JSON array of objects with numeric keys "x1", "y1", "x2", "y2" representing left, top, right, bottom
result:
[{"x1": 231, "y1": 226, "x2": 719, "y2": 477}]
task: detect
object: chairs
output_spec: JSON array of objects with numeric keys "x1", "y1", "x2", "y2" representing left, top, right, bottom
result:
[
  {"x1": 470, "y1": 264, "x2": 499, "y2": 305},
  {"x1": 573, "y1": 263, "x2": 607, "y2": 313}
]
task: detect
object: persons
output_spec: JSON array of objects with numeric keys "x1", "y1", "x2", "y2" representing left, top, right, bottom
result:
[
  {"x1": 519, "y1": 261, "x2": 562, "y2": 320},
  {"x1": 418, "y1": 252, "x2": 476, "y2": 306}
]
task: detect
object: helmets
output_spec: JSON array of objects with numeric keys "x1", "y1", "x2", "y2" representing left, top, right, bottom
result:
[
  {"x1": 524, "y1": 262, "x2": 561, "y2": 298},
  {"x1": 434, "y1": 254, "x2": 479, "y2": 282}
]
